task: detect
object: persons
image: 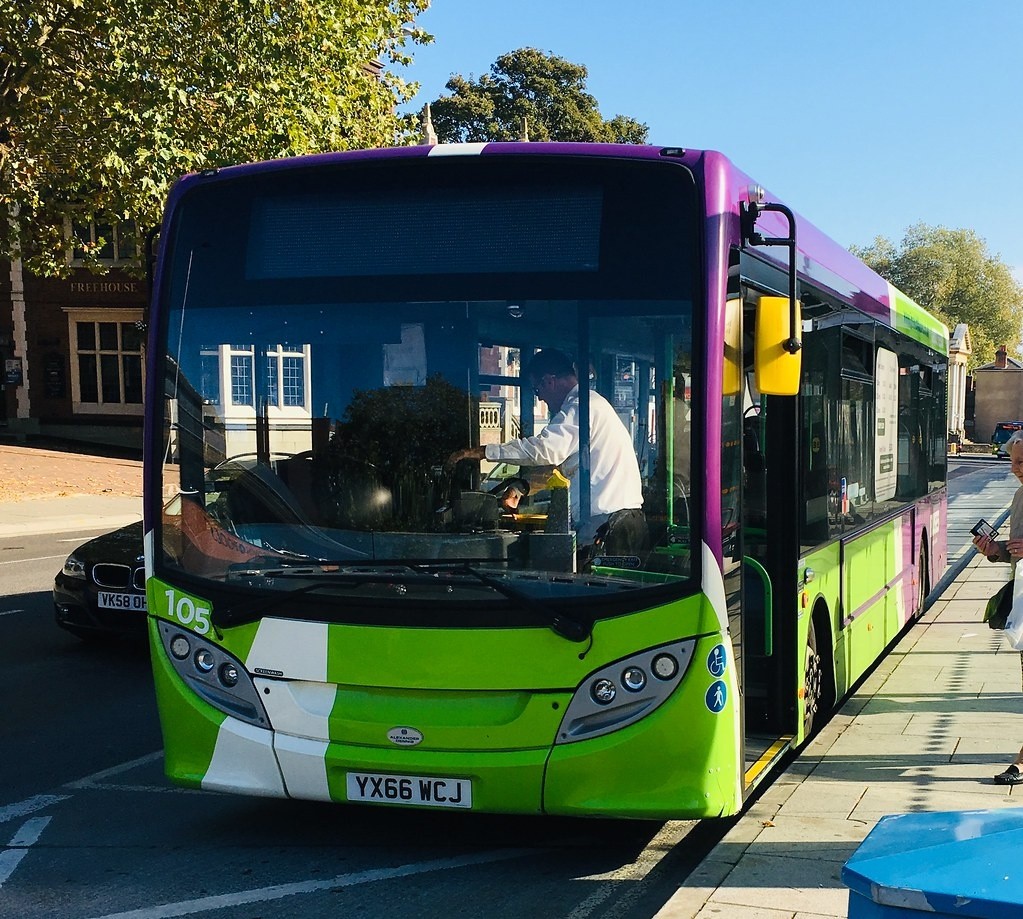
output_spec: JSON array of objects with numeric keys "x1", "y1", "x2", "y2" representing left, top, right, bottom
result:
[
  {"x1": 972, "y1": 429, "x2": 1023, "y2": 785},
  {"x1": 673, "y1": 369, "x2": 691, "y2": 525},
  {"x1": 447, "y1": 348, "x2": 644, "y2": 560}
]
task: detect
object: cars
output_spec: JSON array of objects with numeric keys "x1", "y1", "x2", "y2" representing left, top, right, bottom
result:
[
  {"x1": 481, "y1": 462, "x2": 554, "y2": 514},
  {"x1": 51, "y1": 450, "x2": 298, "y2": 660}
]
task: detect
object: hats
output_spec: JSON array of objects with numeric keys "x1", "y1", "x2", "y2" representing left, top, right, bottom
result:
[{"x1": 531, "y1": 348, "x2": 569, "y2": 374}]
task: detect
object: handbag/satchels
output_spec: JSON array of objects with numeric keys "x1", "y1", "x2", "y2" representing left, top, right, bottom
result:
[
  {"x1": 1004, "y1": 559, "x2": 1023, "y2": 650},
  {"x1": 983, "y1": 579, "x2": 1014, "y2": 629}
]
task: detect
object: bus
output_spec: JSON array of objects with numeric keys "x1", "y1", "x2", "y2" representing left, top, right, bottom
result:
[
  {"x1": 141, "y1": 144, "x2": 952, "y2": 823},
  {"x1": 990, "y1": 421, "x2": 1023, "y2": 460}
]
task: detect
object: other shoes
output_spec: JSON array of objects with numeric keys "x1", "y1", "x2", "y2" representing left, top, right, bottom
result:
[{"x1": 994, "y1": 765, "x2": 1023, "y2": 784}]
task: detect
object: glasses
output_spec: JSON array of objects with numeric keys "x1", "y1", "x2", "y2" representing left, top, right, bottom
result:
[{"x1": 530, "y1": 375, "x2": 556, "y2": 395}]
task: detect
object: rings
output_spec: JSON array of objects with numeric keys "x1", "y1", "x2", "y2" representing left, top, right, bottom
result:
[{"x1": 1014, "y1": 549, "x2": 1017, "y2": 554}]
